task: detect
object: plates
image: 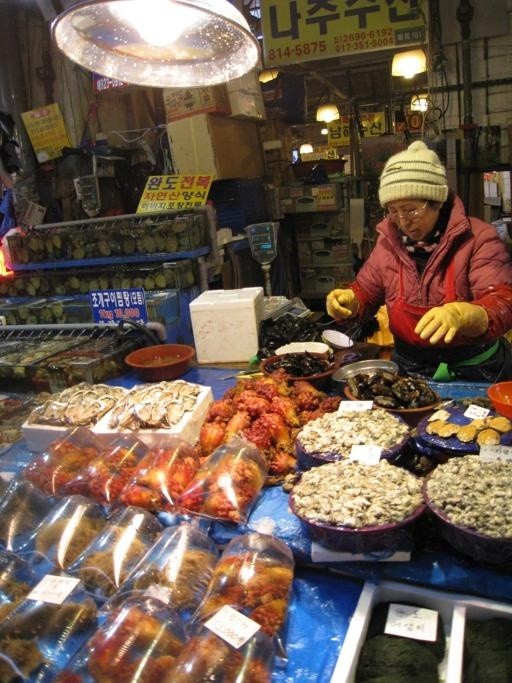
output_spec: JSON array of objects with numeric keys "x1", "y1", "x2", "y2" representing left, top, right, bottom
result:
[
  {"x1": 322, "y1": 329, "x2": 353, "y2": 348},
  {"x1": 418, "y1": 401, "x2": 512, "y2": 457}
]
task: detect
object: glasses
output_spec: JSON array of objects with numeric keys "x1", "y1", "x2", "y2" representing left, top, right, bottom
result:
[{"x1": 383, "y1": 200, "x2": 429, "y2": 223}]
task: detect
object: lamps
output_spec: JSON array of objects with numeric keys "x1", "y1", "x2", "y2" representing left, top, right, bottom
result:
[
  {"x1": 315, "y1": 63, "x2": 340, "y2": 121},
  {"x1": 390, "y1": 50, "x2": 433, "y2": 114},
  {"x1": 45, "y1": 0, "x2": 260, "y2": 90}
]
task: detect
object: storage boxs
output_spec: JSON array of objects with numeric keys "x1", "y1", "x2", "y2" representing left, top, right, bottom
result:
[
  {"x1": 171, "y1": 117, "x2": 265, "y2": 179},
  {"x1": 164, "y1": 84, "x2": 232, "y2": 116},
  {"x1": 188, "y1": 283, "x2": 265, "y2": 363}
]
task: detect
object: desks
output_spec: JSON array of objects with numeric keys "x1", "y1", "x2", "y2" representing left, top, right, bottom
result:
[{"x1": 1, "y1": 365, "x2": 512, "y2": 682}]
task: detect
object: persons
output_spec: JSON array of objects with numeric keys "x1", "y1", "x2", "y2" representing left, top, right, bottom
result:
[{"x1": 325, "y1": 139, "x2": 512, "y2": 385}]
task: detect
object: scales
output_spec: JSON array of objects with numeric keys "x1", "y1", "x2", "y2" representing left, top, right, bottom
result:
[{"x1": 245, "y1": 222, "x2": 315, "y2": 322}]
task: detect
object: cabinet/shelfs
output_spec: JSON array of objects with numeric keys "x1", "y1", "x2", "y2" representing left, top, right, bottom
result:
[
  {"x1": 0, "y1": 212, "x2": 210, "y2": 326},
  {"x1": 278, "y1": 174, "x2": 353, "y2": 298}
]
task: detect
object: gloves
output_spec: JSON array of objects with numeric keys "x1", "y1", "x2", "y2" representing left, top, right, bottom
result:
[
  {"x1": 326, "y1": 288, "x2": 360, "y2": 319},
  {"x1": 414, "y1": 302, "x2": 489, "y2": 345}
]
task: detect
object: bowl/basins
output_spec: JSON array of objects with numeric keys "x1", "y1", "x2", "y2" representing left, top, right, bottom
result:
[
  {"x1": 293, "y1": 411, "x2": 413, "y2": 471},
  {"x1": 423, "y1": 474, "x2": 512, "y2": 568},
  {"x1": 286, "y1": 483, "x2": 428, "y2": 553},
  {"x1": 124, "y1": 344, "x2": 195, "y2": 385},
  {"x1": 290, "y1": 159, "x2": 350, "y2": 180},
  {"x1": 488, "y1": 381, "x2": 512, "y2": 418},
  {"x1": 260, "y1": 351, "x2": 342, "y2": 389},
  {"x1": 333, "y1": 358, "x2": 398, "y2": 395},
  {"x1": 343, "y1": 380, "x2": 438, "y2": 425}
]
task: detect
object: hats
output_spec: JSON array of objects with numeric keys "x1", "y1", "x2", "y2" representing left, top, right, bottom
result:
[{"x1": 378, "y1": 140, "x2": 449, "y2": 210}]
